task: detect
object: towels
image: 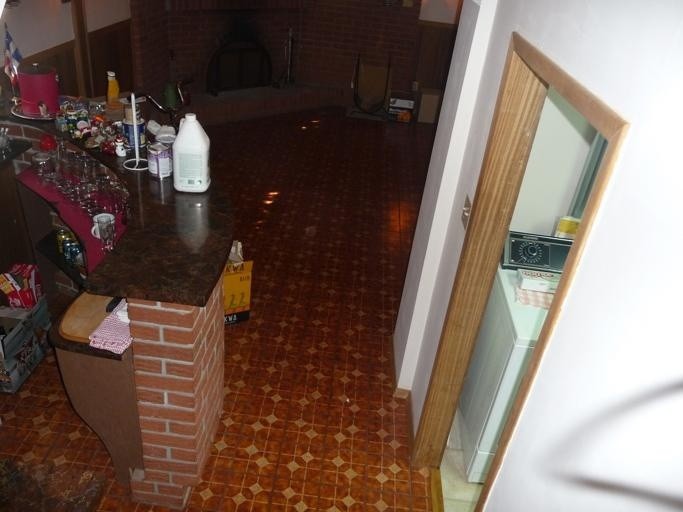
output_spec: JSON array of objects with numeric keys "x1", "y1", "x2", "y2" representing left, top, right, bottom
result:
[
  {"x1": 89, "y1": 299, "x2": 131, "y2": 347},
  {"x1": 89, "y1": 337, "x2": 132, "y2": 354}
]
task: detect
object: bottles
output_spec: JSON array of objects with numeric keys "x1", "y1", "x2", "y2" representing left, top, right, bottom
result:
[
  {"x1": 55, "y1": 227, "x2": 87, "y2": 280},
  {"x1": 122, "y1": 118, "x2": 146, "y2": 149},
  {"x1": 146, "y1": 143, "x2": 173, "y2": 178}
]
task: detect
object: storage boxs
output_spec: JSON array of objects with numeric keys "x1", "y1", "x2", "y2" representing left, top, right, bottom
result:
[
  {"x1": 387, "y1": 93, "x2": 414, "y2": 126},
  {"x1": 0, "y1": 315, "x2": 44, "y2": 399},
  {"x1": 0, "y1": 290, "x2": 56, "y2": 356},
  {"x1": 414, "y1": 85, "x2": 443, "y2": 124},
  {"x1": 221, "y1": 261, "x2": 255, "y2": 327}
]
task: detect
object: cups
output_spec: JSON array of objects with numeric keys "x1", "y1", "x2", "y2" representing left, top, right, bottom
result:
[
  {"x1": 91, "y1": 213, "x2": 115, "y2": 239},
  {"x1": 97, "y1": 215, "x2": 115, "y2": 254},
  {"x1": 30, "y1": 139, "x2": 129, "y2": 217}
]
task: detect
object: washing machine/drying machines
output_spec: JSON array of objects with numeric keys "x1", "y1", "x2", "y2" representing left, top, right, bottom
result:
[{"x1": 457, "y1": 230, "x2": 575, "y2": 484}]
